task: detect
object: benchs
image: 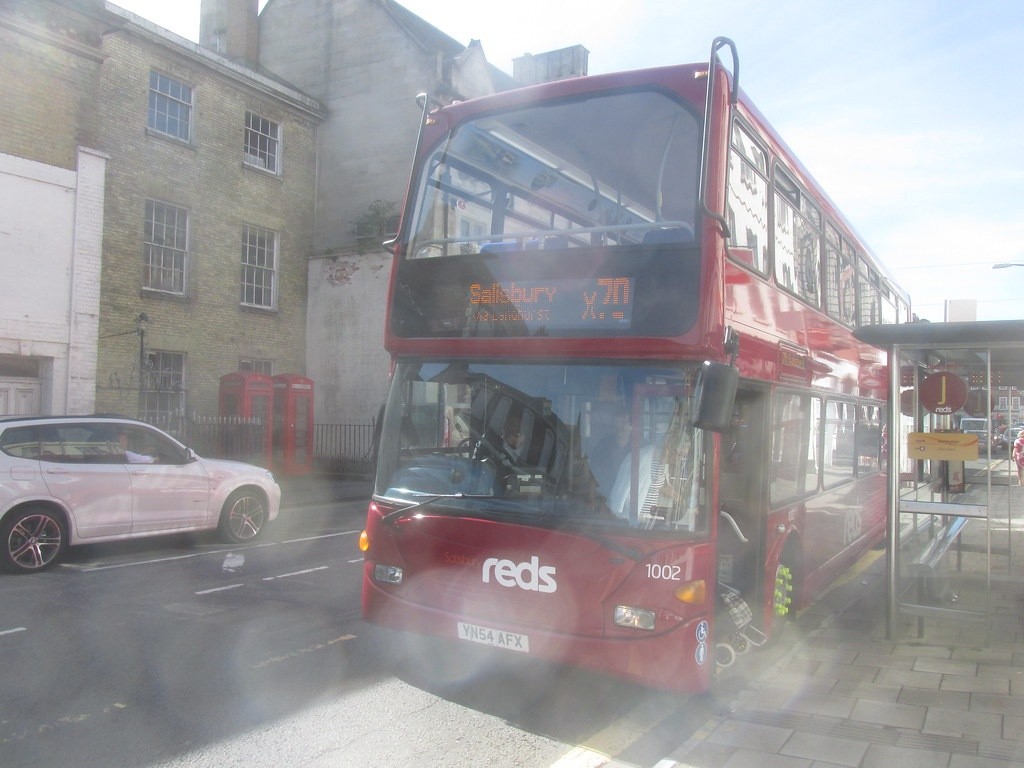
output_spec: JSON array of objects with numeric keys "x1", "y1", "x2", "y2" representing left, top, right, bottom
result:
[{"x1": 907, "y1": 516, "x2": 969, "y2": 641}]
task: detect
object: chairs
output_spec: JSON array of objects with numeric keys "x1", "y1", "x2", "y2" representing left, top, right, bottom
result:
[{"x1": 474, "y1": 227, "x2": 694, "y2": 243}]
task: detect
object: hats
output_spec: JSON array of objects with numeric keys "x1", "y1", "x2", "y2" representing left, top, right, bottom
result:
[{"x1": 1016, "y1": 431, "x2": 1024, "y2": 437}]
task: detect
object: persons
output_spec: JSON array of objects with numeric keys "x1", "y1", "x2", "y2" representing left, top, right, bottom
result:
[
  {"x1": 588, "y1": 406, "x2": 691, "y2": 528},
  {"x1": 1012, "y1": 431, "x2": 1024, "y2": 484},
  {"x1": 117, "y1": 431, "x2": 160, "y2": 463},
  {"x1": 474, "y1": 413, "x2": 531, "y2": 500}
]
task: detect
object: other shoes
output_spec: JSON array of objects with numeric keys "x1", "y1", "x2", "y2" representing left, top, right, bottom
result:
[
  {"x1": 1019, "y1": 480, "x2": 1022, "y2": 484},
  {"x1": 1021, "y1": 483, "x2": 1024, "y2": 487}
]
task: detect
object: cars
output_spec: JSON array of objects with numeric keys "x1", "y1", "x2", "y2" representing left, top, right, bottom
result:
[{"x1": 959, "y1": 417, "x2": 1024, "y2": 454}]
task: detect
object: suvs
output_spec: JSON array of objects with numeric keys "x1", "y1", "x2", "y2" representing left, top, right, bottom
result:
[{"x1": 0, "y1": 413, "x2": 282, "y2": 576}]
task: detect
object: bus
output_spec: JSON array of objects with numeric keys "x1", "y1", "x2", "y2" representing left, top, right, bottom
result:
[{"x1": 357, "y1": 33, "x2": 913, "y2": 701}]
